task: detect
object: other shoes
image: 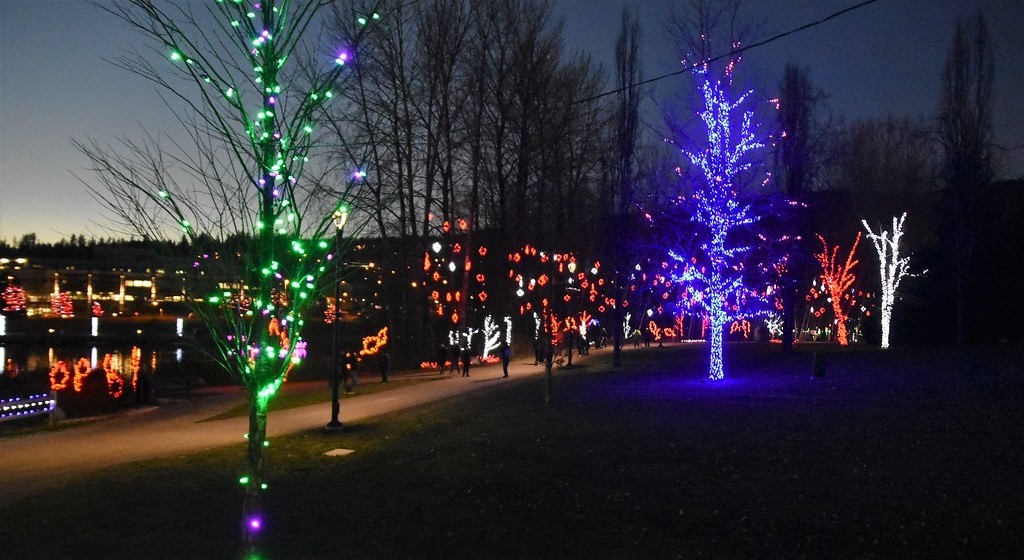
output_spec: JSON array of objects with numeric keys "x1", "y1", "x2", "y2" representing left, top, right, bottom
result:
[
  {"x1": 536, "y1": 360, "x2": 541, "y2": 365},
  {"x1": 503, "y1": 374, "x2": 508, "y2": 377},
  {"x1": 343, "y1": 391, "x2": 355, "y2": 395},
  {"x1": 635, "y1": 346, "x2": 641, "y2": 348},
  {"x1": 353, "y1": 382, "x2": 360, "y2": 386},
  {"x1": 455, "y1": 372, "x2": 460, "y2": 376},
  {"x1": 463, "y1": 375, "x2": 470, "y2": 377},
  {"x1": 381, "y1": 381, "x2": 388, "y2": 384},
  {"x1": 578, "y1": 353, "x2": 582, "y2": 356},
  {"x1": 449, "y1": 372, "x2": 453, "y2": 376},
  {"x1": 600, "y1": 345, "x2": 607, "y2": 348},
  {"x1": 439, "y1": 373, "x2": 444, "y2": 376},
  {"x1": 645, "y1": 345, "x2": 649, "y2": 347}
]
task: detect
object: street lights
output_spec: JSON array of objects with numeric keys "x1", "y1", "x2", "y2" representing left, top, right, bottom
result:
[{"x1": 325, "y1": 206, "x2": 347, "y2": 431}]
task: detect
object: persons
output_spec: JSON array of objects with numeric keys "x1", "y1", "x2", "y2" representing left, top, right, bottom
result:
[{"x1": 339, "y1": 326, "x2": 665, "y2": 395}]
